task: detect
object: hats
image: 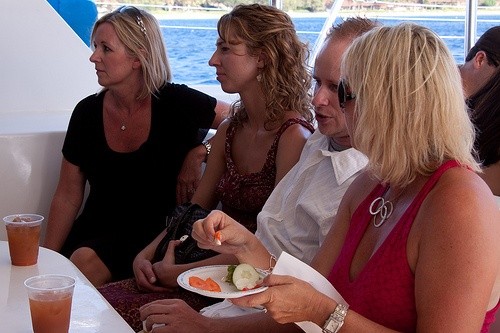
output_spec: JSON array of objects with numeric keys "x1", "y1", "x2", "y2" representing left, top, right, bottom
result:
[{"x1": 475, "y1": 26, "x2": 500, "y2": 66}]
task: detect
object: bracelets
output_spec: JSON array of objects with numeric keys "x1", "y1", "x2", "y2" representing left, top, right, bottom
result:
[
  {"x1": 268, "y1": 251, "x2": 277, "y2": 269},
  {"x1": 202, "y1": 140, "x2": 212, "y2": 154}
]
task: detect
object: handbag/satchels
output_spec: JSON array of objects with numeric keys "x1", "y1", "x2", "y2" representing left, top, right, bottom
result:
[{"x1": 152, "y1": 204, "x2": 220, "y2": 265}]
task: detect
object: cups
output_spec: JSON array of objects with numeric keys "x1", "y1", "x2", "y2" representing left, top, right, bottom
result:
[
  {"x1": 24, "y1": 274, "x2": 76, "y2": 333},
  {"x1": 2, "y1": 214, "x2": 44, "y2": 266}
]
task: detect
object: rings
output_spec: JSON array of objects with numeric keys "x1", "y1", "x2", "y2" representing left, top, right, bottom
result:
[{"x1": 263, "y1": 307, "x2": 267, "y2": 314}]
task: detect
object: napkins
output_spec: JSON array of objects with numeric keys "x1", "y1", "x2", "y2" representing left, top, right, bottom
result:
[{"x1": 272, "y1": 251, "x2": 350, "y2": 333}]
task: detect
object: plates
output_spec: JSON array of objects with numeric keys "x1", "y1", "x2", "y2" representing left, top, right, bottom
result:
[{"x1": 176, "y1": 264, "x2": 270, "y2": 299}]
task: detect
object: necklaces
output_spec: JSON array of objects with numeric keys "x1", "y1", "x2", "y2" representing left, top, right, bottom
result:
[
  {"x1": 369, "y1": 185, "x2": 409, "y2": 228},
  {"x1": 116, "y1": 98, "x2": 138, "y2": 131}
]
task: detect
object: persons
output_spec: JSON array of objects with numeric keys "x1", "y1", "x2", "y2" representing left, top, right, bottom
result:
[
  {"x1": 140, "y1": 19, "x2": 500, "y2": 333},
  {"x1": 43, "y1": 6, "x2": 239, "y2": 285},
  {"x1": 99, "y1": 5, "x2": 314, "y2": 333}
]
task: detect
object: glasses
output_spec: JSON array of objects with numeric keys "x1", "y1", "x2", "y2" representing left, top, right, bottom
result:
[
  {"x1": 337, "y1": 81, "x2": 357, "y2": 107},
  {"x1": 116, "y1": 5, "x2": 146, "y2": 35}
]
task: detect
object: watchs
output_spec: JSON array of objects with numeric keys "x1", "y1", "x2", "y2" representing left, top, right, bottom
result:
[{"x1": 322, "y1": 304, "x2": 348, "y2": 333}]
